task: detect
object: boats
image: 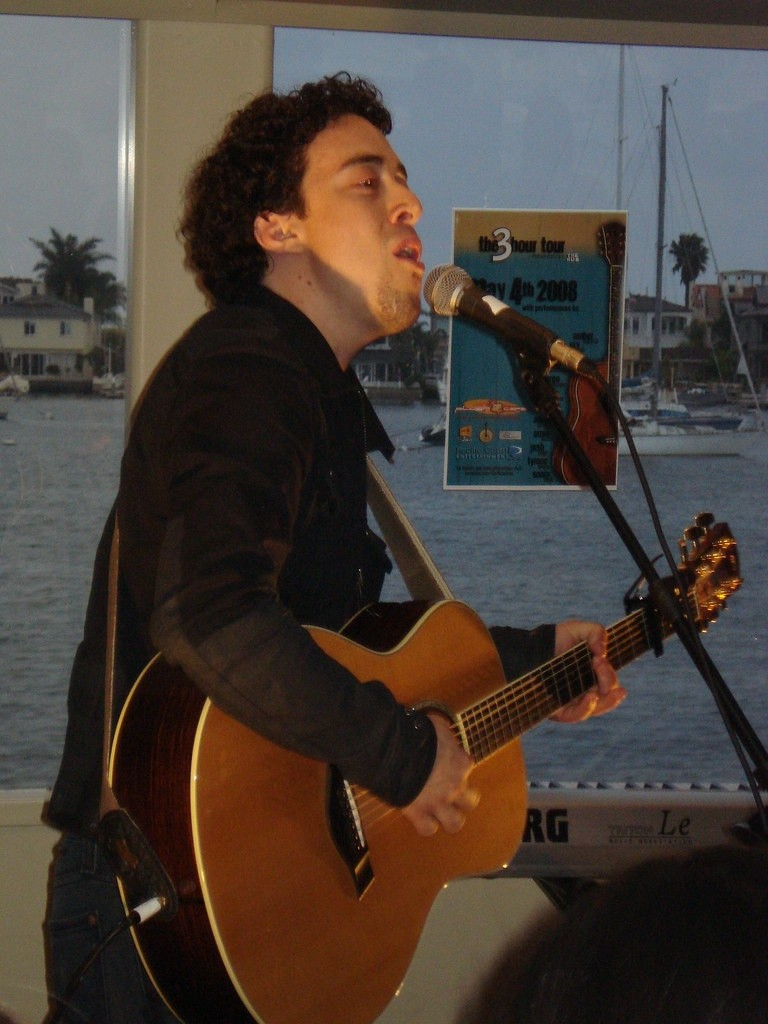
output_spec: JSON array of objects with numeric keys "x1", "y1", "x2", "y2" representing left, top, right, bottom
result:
[
  {"x1": 0, "y1": 372, "x2": 33, "y2": 398},
  {"x1": 89, "y1": 371, "x2": 125, "y2": 400},
  {"x1": 418, "y1": 415, "x2": 448, "y2": 446}
]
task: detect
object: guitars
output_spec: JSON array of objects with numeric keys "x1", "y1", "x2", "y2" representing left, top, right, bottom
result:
[
  {"x1": 104, "y1": 510, "x2": 746, "y2": 1024},
  {"x1": 554, "y1": 216, "x2": 626, "y2": 486}
]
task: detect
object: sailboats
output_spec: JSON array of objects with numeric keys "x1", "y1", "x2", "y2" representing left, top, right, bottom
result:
[{"x1": 615, "y1": 83, "x2": 763, "y2": 457}]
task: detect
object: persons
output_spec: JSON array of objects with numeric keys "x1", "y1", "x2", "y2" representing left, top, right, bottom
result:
[{"x1": 41, "y1": 71, "x2": 629, "y2": 1024}]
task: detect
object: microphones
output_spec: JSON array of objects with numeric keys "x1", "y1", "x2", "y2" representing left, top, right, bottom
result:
[{"x1": 423, "y1": 264, "x2": 597, "y2": 374}]
